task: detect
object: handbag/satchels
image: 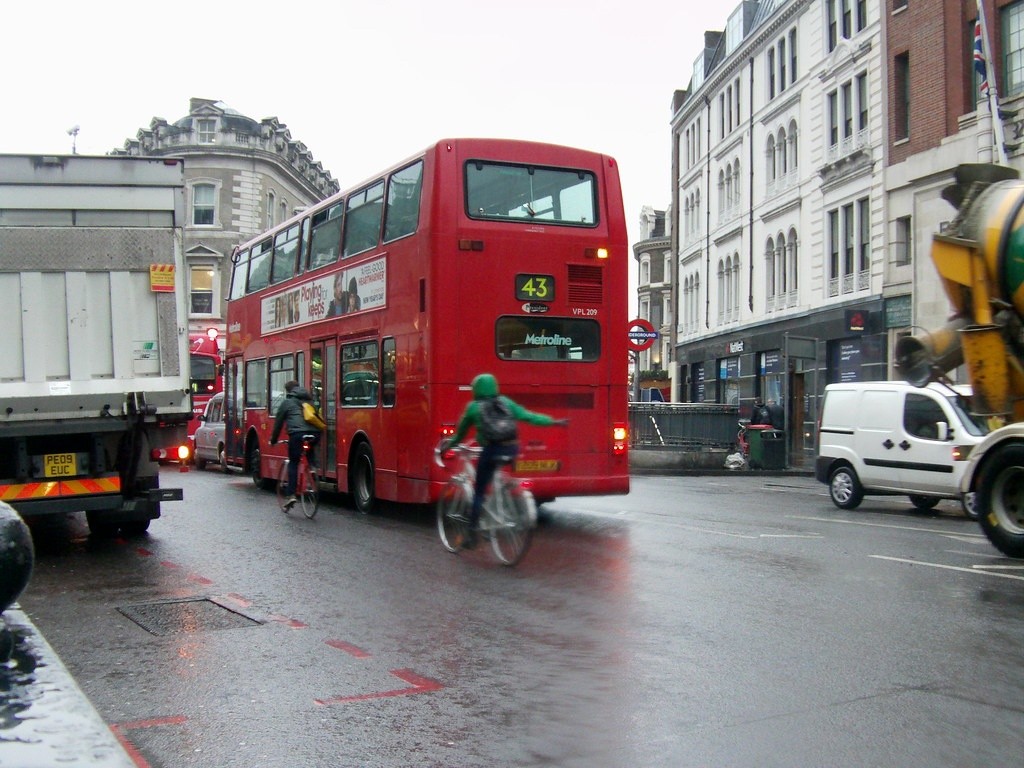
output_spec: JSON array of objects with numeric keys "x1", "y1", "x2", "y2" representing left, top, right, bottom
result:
[{"x1": 302, "y1": 402, "x2": 327, "y2": 431}]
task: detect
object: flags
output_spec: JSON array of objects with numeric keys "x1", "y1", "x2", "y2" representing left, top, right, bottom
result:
[{"x1": 973, "y1": 0, "x2": 1002, "y2": 117}]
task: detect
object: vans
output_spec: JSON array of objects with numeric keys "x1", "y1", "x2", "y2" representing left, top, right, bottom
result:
[{"x1": 813, "y1": 379, "x2": 990, "y2": 522}]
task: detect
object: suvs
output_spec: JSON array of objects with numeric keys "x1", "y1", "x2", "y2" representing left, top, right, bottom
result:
[{"x1": 194, "y1": 391, "x2": 284, "y2": 473}]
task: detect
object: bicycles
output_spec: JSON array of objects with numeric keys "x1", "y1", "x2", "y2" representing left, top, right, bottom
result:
[
  {"x1": 268, "y1": 434, "x2": 321, "y2": 520},
  {"x1": 432, "y1": 439, "x2": 539, "y2": 568}
]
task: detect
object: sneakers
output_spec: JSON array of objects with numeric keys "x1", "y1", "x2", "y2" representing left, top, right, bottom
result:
[
  {"x1": 309, "y1": 466, "x2": 317, "y2": 474},
  {"x1": 282, "y1": 495, "x2": 297, "y2": 508}
]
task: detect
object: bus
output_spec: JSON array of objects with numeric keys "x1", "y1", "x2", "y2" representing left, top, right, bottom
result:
[
  {"x1": 150, "y1": 334, "x2": 223, "y2": 464},
  {"x1": 216, "y1": 136, "x2": 631, "y2": 516}
]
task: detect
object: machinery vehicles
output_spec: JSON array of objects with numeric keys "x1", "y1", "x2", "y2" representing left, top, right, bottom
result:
[{"x1": 895, "y1": 162, "x2": 1024, "y2": 567}]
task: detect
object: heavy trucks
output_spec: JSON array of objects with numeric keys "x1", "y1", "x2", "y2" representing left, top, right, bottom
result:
[{"x1": 1, "y1": 151, "x2": 197, "y2": 547}]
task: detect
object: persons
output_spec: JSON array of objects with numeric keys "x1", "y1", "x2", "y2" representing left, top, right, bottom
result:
[
  {"x1": 274, "y1": 270, "x2": 361, "y2": 328},
  {"x1": 445, "y1": 374, "x2": 571, "y2": 553},
  {"x1": 271, "y1": 381, "x2": 320, "y2": 507}
]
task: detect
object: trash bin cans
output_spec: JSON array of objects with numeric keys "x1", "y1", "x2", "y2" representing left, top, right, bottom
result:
[
  {"x1": 760, "y1": 429, "x2": 785, "y2": 471},
  {"x1": 744, "y1": 424, "x2": 774, "y2": 468}
]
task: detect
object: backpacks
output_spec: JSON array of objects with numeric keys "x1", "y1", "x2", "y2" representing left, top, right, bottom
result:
[{"x1": 475, "y1": 398, "x2": 518, "y2": 446}]
